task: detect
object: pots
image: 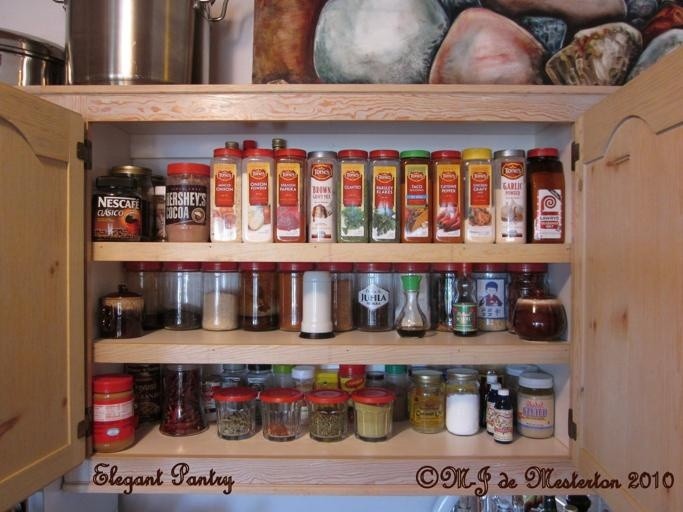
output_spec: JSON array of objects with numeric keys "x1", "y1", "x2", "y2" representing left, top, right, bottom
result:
[
  {"x1": 48, "y1": 0, "x2": 232, "y2": 86},
  {"x1": 0, "y1": 24, "x2": 65, "y2": 87}
]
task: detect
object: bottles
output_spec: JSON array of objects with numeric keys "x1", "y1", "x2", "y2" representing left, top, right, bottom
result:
[
  {"x1": 96, "y1": 259, "x2": 568, "y2": 345},
  {"x1": 91, "y1": 363, "x2": 558, "y2": 454},
  {"x1": 90, "y1": 137, "x2": 567, "y2": 243}
]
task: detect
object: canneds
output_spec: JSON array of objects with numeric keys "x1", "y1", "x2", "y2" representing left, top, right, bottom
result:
[
  {"x1": 91, "y1": 162, "x2": 210, "y2": 243},
  {"x1": 408, "y1": 364, "x2": 555, "y2": 440},
  {"x1": 92, "y1": 362, "x2": 161, "y2": 453}
]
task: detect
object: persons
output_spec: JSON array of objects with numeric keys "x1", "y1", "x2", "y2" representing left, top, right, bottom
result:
[{"x1": 479, "y1": 282, "x2": 503, "y2": 307}]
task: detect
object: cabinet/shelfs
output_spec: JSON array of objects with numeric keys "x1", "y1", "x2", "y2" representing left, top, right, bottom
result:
[{"x1": 0, "y1": 40, "x2": 683, "y2": 512}]
